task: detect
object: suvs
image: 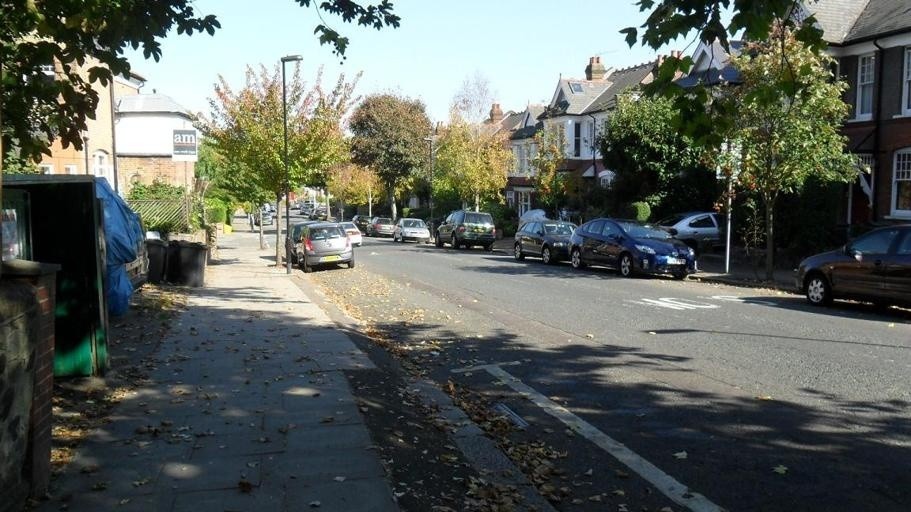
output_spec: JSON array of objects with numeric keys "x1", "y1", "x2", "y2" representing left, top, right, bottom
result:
[{"x1": 434, "y1": 206, "x2": 499, "y2": 251}]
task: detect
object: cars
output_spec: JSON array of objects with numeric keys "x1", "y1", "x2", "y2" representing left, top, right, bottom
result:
[
  {"x1": 391, "y1": 216, "x2": 431, "y2": 244},
  {"x1": 568, "y1": 215, "x2": 700, "y2": 283},
  {"x1": 251, "y1": 198, "x2": 397, "y2": 263},
  {"x1": 294, "y1": 220, "x2": 355, "y2": 272},
  {"x1": 513, "y1": 219, "x2": 580, "y2": 267},
  {"x1": 653, "y1": 208, "x2": 735, "y2": 253},
  {"x1": 790, "y1": 223, "x2": 911, "y2": 314}
]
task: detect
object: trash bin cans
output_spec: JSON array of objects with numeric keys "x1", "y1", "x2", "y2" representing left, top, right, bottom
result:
[{"x1": 146, "y1": 238, "x2": 207, "y2": 286}]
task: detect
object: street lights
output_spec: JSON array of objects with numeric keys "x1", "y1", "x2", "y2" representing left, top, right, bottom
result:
[
  {"x1": 422, "y1": 137, "x2": 437, "y2": 244},
  {"x1": 281, "y1": 52, "x2": 307, "y2": 276}
]
